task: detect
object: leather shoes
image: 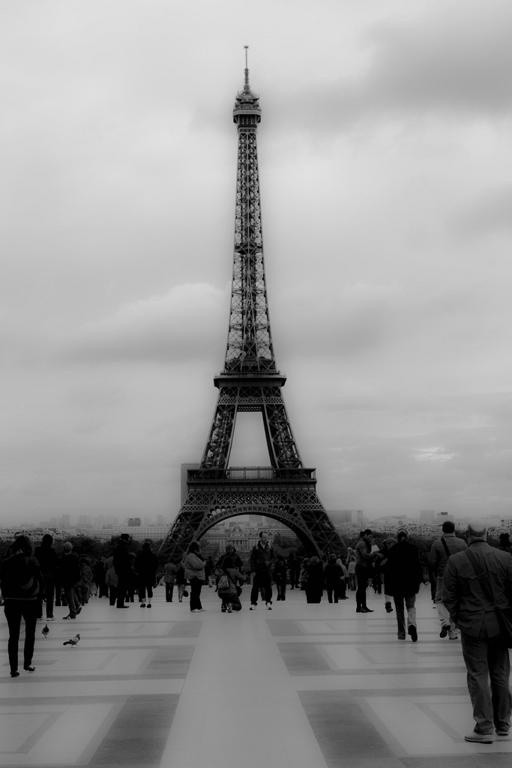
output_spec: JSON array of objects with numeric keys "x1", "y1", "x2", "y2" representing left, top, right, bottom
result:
[
  {"x1": 440, "y1": 624, "x2": 450, "y2": 638},
  {"x1": 363, "y1": 607, "x2": 374, "y2": 612},
  {"x1": 464, "y1": 734, "x2": 494, "y2": 742},
  {"x1": 117, "y1": 604, "x2": 152, "y2": 608},
  {"x1": 356, "y1": 607, "x2": 366, "y2": 613},
  {"x1": 409, "y1": 625, "x2": 417, "y2": 642},
  {"x1": 24, "y1": 665, "x2": 35, "y2": 671}
]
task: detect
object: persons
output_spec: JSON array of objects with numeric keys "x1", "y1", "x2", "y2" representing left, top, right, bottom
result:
[
  {"x1": 383, "y1": 529, "x2": 425, "y2": 641},
  {"x1": 1, "y1": 519, "x2": 512, "y2": 613},
  {"x1": 34, "y1": 534, "x2": 56, "y2": 620},
  {"x1": 58, "y1": 542, "x2": 82, "y2": 620},
  {"x1": 430, "y1": 521, "x2": 467, "y2": 639},
  {"x1": 441, "y1": 522, "x2": 511, "y2": 743},
  {"x1": 1, "y1": 535, "x2": 39, "y2": 677}
]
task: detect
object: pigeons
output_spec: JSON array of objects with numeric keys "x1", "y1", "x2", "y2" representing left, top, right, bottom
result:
[
  {"x1": 41, "y1": 624, "x2": 50, "y2": 640},
  {"x1": 63, "y1": 633, "x2": 80, "y2": 646}
]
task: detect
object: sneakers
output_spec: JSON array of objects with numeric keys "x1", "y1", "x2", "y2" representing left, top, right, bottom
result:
[
  {"x1": 267, "y1": 601, "x2": 272, "y2": 610},
  {"x1": 191, "y1": 602, "x2": 233, "y2": 614},
  {"x1": 249, "y1": 604, "x2": 255, "y2": 610}
]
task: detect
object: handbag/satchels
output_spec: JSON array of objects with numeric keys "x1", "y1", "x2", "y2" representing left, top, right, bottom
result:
[
  {"x1": 494, "y1": 605, "x2": 512, "y2": 648},
  {"x1": 217, "y1": 573, "x2": 232, "y2": 600}
]
